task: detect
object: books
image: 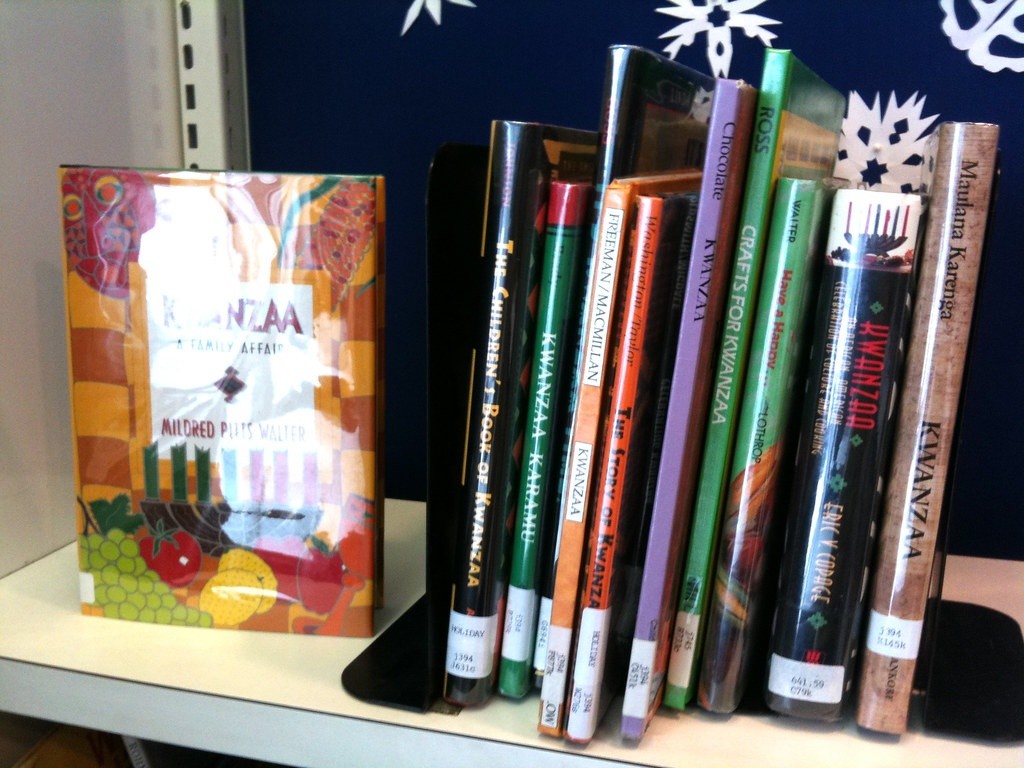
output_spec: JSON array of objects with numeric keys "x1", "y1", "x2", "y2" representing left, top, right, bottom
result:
[
  {"x1": 55, "y1": 162, "x2": 389, "y2": 638},
  {"x1": 439, "y1": 45, "x2": 998, "y2": 745}
]
